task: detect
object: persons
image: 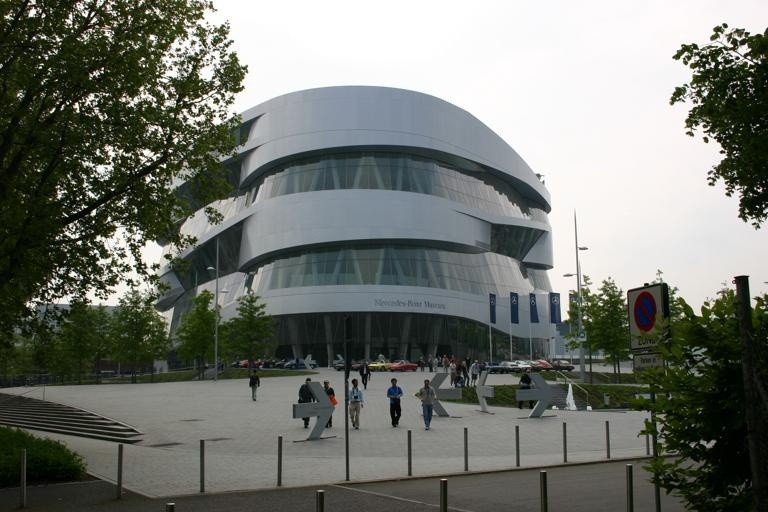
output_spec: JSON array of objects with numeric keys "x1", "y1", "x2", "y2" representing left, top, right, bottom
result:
[
  {"x1": 387, "y1": 378, "x2": 403, "y2": 427},
  {"x1": 517, "y1": 369, "x2": 534, "y2": 410},
  {"x1": 415, "y1": 379, "x2": 437, "y2": 430},
  {"x1": 359, "y1": 362, "x2": 371, "y2": 389},
  {"x1": 298, "y1": 378, "x2": 315, "y2": 428},
  {"x1": 349, "y1": 378, "x2": 364, "y2": 430},
  {"x1": 418, "y1": 352, "x2": 488, "y2": 388},
  {"x1": 217, "y1": 355, "x2": 241, "y2": 372},
  {"x1": 322, "y1": 380, "x2": 335, "y2": 428},
  {"x1": 249, "y1": 369, "x2": 260, "y2": 401},
  {"x1": 256, "y1": 355, "x2": 300, "y2": 370}
]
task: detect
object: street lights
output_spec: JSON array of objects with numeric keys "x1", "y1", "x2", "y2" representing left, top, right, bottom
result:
[
  {"x1": 206, "y1": 238, "x2": 228, "y2": 380},
  {"x1": 563, "y1": 209, "x2": 588, "y2": 379}
]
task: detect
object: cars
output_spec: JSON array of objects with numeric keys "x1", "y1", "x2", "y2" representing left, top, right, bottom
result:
[
  {"x1": 484, "y1": 360, "x2": 574, "y2": 373},
  {"x1": 335, "y1": 360, "x2": 418, "y2": 373},
  {"x1": 237, "y1": 360, "x2": 317, "y2": 369}
]
